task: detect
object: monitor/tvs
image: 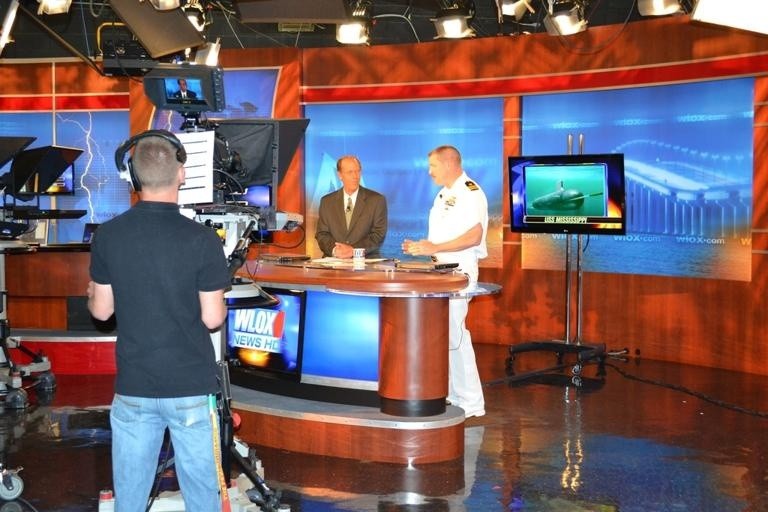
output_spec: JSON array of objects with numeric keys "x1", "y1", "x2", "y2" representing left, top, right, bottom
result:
[
  {"x1": 239, "y1": 185, "x2": 272, "y2": 243},
  {"x1": 226, "y1": 286, "x2": 307, "y2": 383},
  {"x1": 16, "y1": 162, "x2": 74, "y2": 195},
  {"x1": 155, "y1": 69, "x2": 216, "y2": 112},
  {"x1": 508, "y1": 153, "x2": 626, "y2": 236}
]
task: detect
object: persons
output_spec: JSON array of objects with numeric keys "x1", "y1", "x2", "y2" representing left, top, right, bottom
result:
[
  {"x1": 86, "y1": 128, "x2": 232, "y2": 512},
  {"x1": 399, "y1": 144, "x2": 487, "y2": 417},
  {"x1": 172, "y1": 78, "x2": 194, "y2": 97},
  {"x1": 314, "y1": 154, "x2": 388, "y2": 257}
]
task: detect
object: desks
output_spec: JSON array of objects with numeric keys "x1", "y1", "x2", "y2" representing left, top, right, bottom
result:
[{"x1": 226, "y1": 256, "x2": 470, "y2": 416}]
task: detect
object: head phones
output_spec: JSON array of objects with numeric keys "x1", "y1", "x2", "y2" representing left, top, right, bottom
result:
[{"x1": 115, "y1": 130, "x2": 187, "y2": 192}]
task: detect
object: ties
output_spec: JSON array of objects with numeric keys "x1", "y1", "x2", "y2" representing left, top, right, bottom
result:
[{"x1": 344, "y1": 197, "x2": 354, "y2": 231}]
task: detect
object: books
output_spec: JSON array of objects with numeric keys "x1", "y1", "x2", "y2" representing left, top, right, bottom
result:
[
  {"x1": 401, "y1": 260, "x2": 459, "y2": 270},
  {"x1": 259, "y1": 252, "x2": 311, "y2": 261}
]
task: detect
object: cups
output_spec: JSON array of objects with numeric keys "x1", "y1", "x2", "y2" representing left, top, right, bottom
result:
[{"x1": 352, "y1": 248, "x2": 364, "y2": 270}]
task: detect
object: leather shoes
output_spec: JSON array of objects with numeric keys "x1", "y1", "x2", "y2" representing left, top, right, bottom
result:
[{"x1": 457, "y1": 405, "x2": 485, "y2": 418}]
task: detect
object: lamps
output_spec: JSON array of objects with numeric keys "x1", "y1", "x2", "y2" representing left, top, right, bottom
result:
[{"x1": 330, "y1": 1, "x2": 684, "y2": 47}]
